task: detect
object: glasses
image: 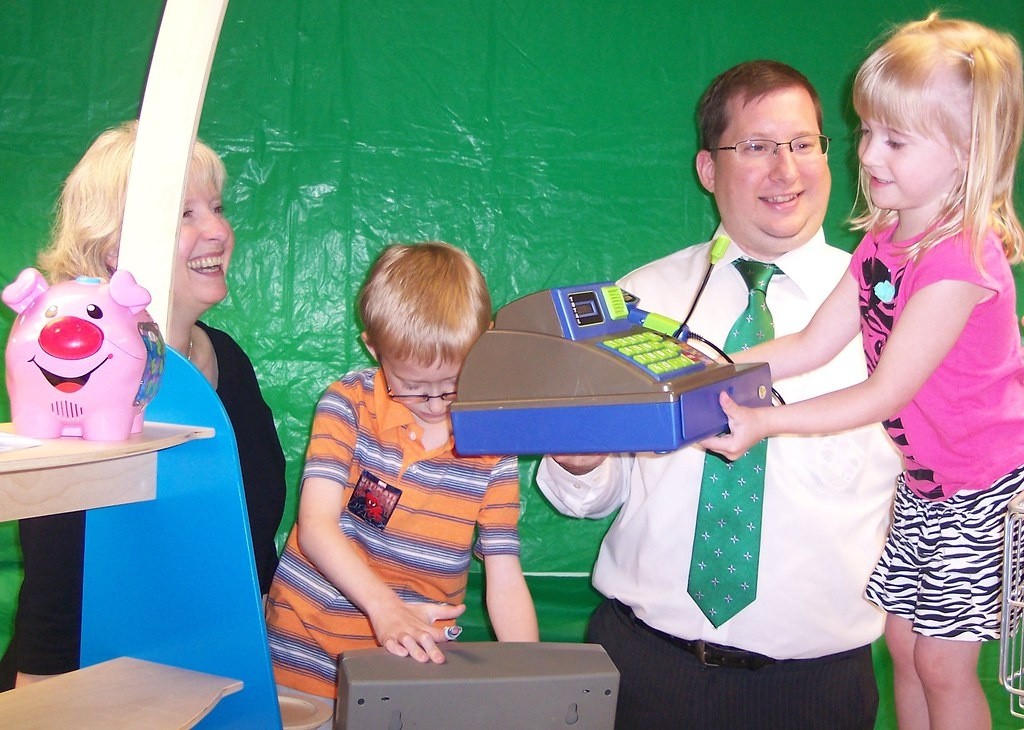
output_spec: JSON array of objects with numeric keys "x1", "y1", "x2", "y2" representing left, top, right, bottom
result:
[
  {"x1": 377, "y1": 353, "x2": 458, "y2": 402},
  {"x1": 704, "y1": 135, "x2": 832, "y2": 161}
]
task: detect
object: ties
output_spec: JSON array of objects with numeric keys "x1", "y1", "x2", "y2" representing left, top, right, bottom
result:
[{"x1": 687, "y1": 258, "x2": 785, "y2": 629}]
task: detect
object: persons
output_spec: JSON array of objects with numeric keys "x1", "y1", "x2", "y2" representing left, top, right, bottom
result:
[
  {"x1": 265, "y1": 239, "x2": 539, "y2": 730},
  {"x1": 702, "y1": 19, "x2": 1024, "y2": 730},
  {"x1": 535, "y1": 60, "x2": 906, "y2": 729},
  {"x1": 8, "y1": 116, "x2": 288, "y2": 690}
]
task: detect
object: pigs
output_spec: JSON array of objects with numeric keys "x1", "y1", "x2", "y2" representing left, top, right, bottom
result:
[{"x1": 2, "y1": 267, "x2": 166, "y2": 443}]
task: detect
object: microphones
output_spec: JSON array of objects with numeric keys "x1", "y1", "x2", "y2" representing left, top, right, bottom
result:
[{"x1": 675, "y1": 234, "x2": 731, "y2": 339}]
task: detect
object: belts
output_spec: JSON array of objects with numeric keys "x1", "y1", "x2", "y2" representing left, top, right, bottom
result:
[{"x1": 611, "y1": 601, "x2": 776, "y2": 672}]
